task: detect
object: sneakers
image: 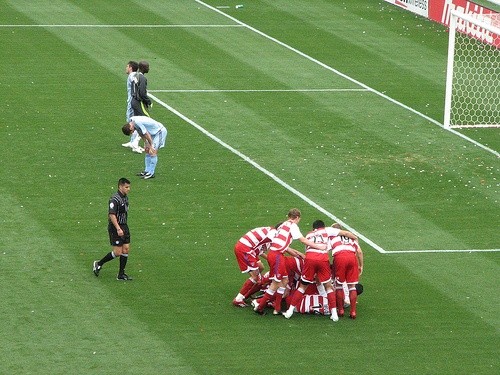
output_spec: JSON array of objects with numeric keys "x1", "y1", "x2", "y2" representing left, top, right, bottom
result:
[
  {"x1": 129, "y1": 142, "x2": 145, "y2": 153},
  {"x1": 350, "y1": 311, "x2": 356, "y2": 319},
  {"x1": 254, "y1": 306, "x2": 267, "y2": 315},
  {"x1": 136, "y1": 171, "x2": 154, "y2": 179},
  {"x1": 232, "y1": 298, "x2": 249, "y2": 308},
  {"x1": 117, "y1": 274, "x2": 132, "y2": 280},
  {"x1": 282, "y1": 310, "x2": 293, "y2": 319},
  {"x1": 93, "y1": 261, "x2": 102, "y2": 277},
  {"x1": 122, "y1": 141, "x2": 134, "y2": 148},
  {"x1": 273, "y1": 309, "x2": 283, "y2": 315},
  {"x1": 330, "y1": 314, "x2": 339, "y2": 322},
  {"x1": 337, "y1": 309, "x2": 344, "y2": 316}
]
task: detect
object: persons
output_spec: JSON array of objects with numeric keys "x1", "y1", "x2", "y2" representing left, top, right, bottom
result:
[
  {"x1": 122, "y1": 116, "x2": 167, "y2": 180},
  {"x1": 93, "y1": 178, "x2": 134, "y2": 281},
  {"x1": 233, "y1": 207, "x2": 364, "y2": 322},
  {"x1": 121, "y1": 61, "x2": 154, "y2": 154}
]
